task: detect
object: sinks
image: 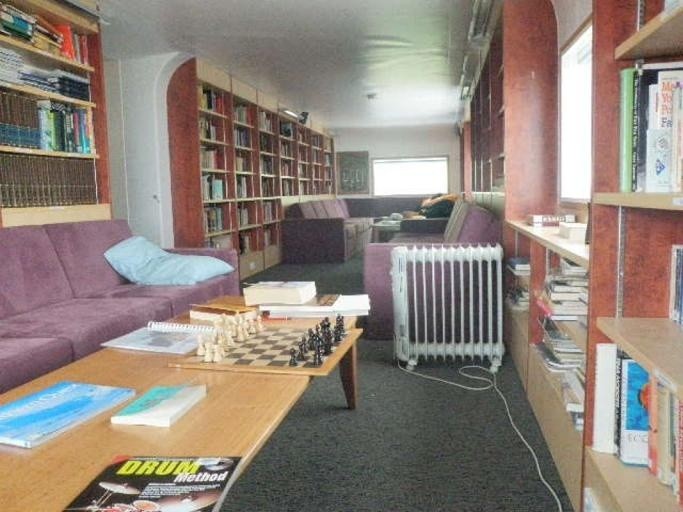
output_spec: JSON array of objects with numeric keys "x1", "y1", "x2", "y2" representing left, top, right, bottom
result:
[
  {"x1": 1, "y1": 217, "x2": 240, "y2": 394},
  {"x1": 363, "y1": 204, "x2": 504, "y2": 341},
  {"x1": 279, "y1": 197, "x2": 374, "y2": 265}
]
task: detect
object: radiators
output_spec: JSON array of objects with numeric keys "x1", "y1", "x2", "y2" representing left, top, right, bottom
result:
[{"x1": 389, "y1": 245, "x2": 505, "y2": 373}]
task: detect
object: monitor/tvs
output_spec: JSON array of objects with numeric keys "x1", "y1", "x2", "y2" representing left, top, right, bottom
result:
[
  {"x1": 0, "y1": 0, "x2": 106, "y2": 215},
  {"x1": 195, "y1": 90, "x2": 335, "y2": 259},
  {"x1": 610, "y1": 49, "x2": 682, "y2": 193},
  {"x1": 0, "y1": 379, "x2": 137, "y2": 450},
  {"x1": 242, "y1": 279, "x2": 317, "y2": 307},
  {"x1": 98, "y1": 321, "x2": 223, "y2": 355},
  {"x1": 188, "y1": 302, "x2": 263, "y2": 324},
  {"x1": 257, "y1": 292, "x2": 371, "y2": 311},
  {"x1": 260, "y1": 289, "x2": 342, "y2": 307},
  {"x1": 268, "y1": 310, "x2": 369, "y2": 319},
  {"x1": 110, "y1": 382, "x2": 208, "y2": 432},
  {"x1": 62, "y1": 452, "x2": 245, "y2": 511}
]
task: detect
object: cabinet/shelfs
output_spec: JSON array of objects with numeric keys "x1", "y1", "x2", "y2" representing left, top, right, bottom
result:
[
  {"x1": 459, "y1": 0, "x2": 683, "y2": 511},
  {"x1": 167, "y1": 57, "x2": 336, "y2": 284},
  {"x1": 0, "y1": 1, "x2": 113, "y2": 227}
]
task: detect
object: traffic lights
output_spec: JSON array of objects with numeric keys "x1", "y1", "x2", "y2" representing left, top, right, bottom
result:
[{"x1": 102, "y1": 235, "x2": 235, "y2": 286}]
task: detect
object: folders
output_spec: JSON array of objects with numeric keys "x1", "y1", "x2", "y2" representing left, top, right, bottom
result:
[{"x1": 389, "y1": 245, "x2": 505, "y2": 373}]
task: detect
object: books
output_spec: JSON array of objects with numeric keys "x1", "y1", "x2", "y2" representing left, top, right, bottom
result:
[
  {"x1": 542, "y1": 257, "x2": 589, "y2": 316},
  {"x1": 591, "y1": 341, "x2": 681, "y2": 502},
  {"x1": 0, "y1": 379, "x2": 137, "y2": 450},
  {"x1": 610, "y1": 49, "x2": 682, "y2": 193},
  {"x1": 535, "y1": 297, "x2": 579, "y2": 321},
  {"x1": 526, "y1": 215, "x2": 576, "y2": 227},
  {"x1": 260, "y1": 289, "x2": 342, "y2": 307},
  {"x1": 508, "y1": 256, "x2": 530, "y2": 271},
  {"x1": 528, "y1": 315, "x2": 585, "y2": 372},
  {"x1": 62, "y1": 452, "x2": 245, "y2": 511},
  {"x1": 257, "y1": 292, "x2": 371, "y2": 311},
  {"x1": 195, "y1": 90, "x2": 335, "y2": 259},
  {"x1": 559, "y1": 222, "x2": 586, "y2": 240},
  {"x1": 188, "y1": 302, "x2": 263, "y2": 324},
  {"x1": 669, "y1": 244, "x2": 682, "y2": 320},
  {"x1": 561, "y1": 368, "x2": 586, "y2": 432},
  {"x1": 98, "y1": 321, "x2": 223, "y2": 355},
  {"x1": 242, "y1": 279, "x2": 317, "y2": 307},
  {"x1": 0, "y1": 0, "x2": 106, "y2": 215},
  {"x1": 268, "y1": 310, "x2": 369, "y2": 319},
  {"x1": 110, "y1": 382, "x2": 208, "y2": 432}
]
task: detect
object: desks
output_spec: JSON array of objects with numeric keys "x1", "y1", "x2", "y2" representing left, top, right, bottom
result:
[{"x1": 1, "y1": 291, "x2": 364, "y2": 510}]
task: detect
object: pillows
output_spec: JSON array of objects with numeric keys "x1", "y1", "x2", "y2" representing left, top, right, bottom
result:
[{"x1": 102, "y1": 235, "x2": 235, "y2": 286}]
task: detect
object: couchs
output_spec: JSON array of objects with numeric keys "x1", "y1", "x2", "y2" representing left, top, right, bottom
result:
[
  {"x1": 1, "y1": 217, "x2": 240, "y2": 394},
  {"x1": 363, "y1": 204, "x2": 504, "y2": 341},
  {"x1": 279, "y1": 197, "x2": 374, "y2": 265}
]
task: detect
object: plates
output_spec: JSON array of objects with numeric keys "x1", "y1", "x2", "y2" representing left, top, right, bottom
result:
[
  {"x1": 167, "y1": 57, "x2": 336, "y2": 284},
  {"x1": 0, "y1": 1, "x2": 113, "y2": 227}
]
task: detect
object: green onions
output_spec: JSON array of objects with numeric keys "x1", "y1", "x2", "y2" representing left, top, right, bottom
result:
[{"x1": 167, "y1": 310, "x2": 365, "y2": 377}]
